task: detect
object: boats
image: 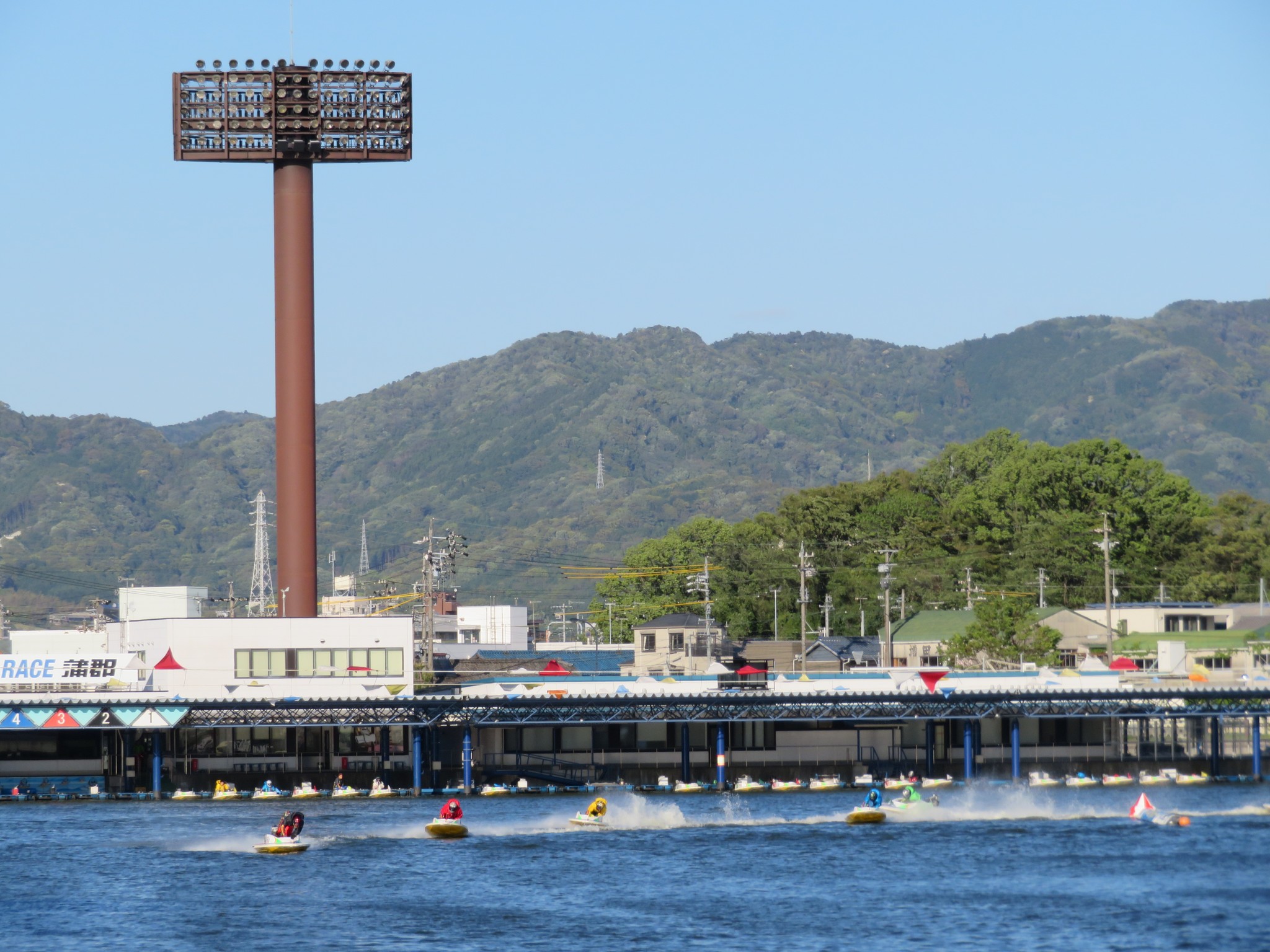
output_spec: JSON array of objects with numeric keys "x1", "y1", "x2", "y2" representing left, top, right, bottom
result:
[
  {"x1": 331, "y1": 788, "x2": 363, "y2": 798},
  {"x1": 171, "y1": 791, "x2": 283, "y2": 801},
  {"x1": 292, "y1": 792, "x2": 322, "y2": 799},
  {"x1": 852, "y1": 782, "x2": 884, "y2": 787},
  {"x1": 921, "y1": 777, "x2": 954, "y2": 789},
  {"x1": 1066, "y1": 777, "x2": 1097, "y2": 787},
  {"x1": 481, "y1": 786, "x2": 510, "y2": 796},
  {"x1": 252, "y1": 834, "x2": 310, "y2": 854},
  {"x1": 892, "y1": 798, "x2": 932, "y2": 810},
  {"x1": 673, "y1": 775, "x2": 771, "y2": 794},
  {"x1": 1139, "y1": 775, "x2": 1170, "y2": 786},
  {"x1": 884, "y1": 779, "x2": 914, "y2": 790},
  {"x1": 369, "y1": 785, "x2": 396, "y2": 799},
  {"x1": 844, "y1": 806, "x2": 887, "y2": 824},
  {"x1": 424, "y1": 817, "x2": 468, "y2": 839},
  {"x1": 569, "y1": 811, "x2": 609, "y2": 829},
  {"x1": 809, "y1": 776, "x2": 846, "y2": 790},
  {"x1": 1176, "y1": 773, "x2": 1206, "y2": 785},
  {"x1": 1102, "y1": 776, "x2": 1133, "y2": 786},
  {"x1": 772, "y1": 781, "x2": 809, "y2": 791},
  {"x1": 1028, "y1": 778, "x2": 1059, "y2": 787}
]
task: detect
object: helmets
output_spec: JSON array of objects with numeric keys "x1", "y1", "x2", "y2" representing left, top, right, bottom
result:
[
  {"x1": 908, "y1": 770, "x2": 915, "y2": 778},
  {"x1": 292, "y1": 816, "x2": 301, "y2": 828},
  {"x1": 596, "y1": 801, "x2": 604, "y2": 810},
  {"x1": 869, "y1": 791, "x2": 878, "y2": 801},
  {"x1": 267, "y1": 780, "x2": 272, "y2": 787},
  {"x1": 902, "y1": 789, "x2": 911, "y2": 799},
  {"x1": 1178, "y1": 817, "x2": 1190, "y2": 827},
  {"x1": 449, "y1": 802, "x2": 457, "y2": 813}
]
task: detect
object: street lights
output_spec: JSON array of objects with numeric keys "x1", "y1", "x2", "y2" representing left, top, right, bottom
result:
[
  {"x1": 769, "y1": 590, "x2": 782, "y2": 641},
  {"x1": 604, "y1": 603, "x2": 616, "y2": 643}
]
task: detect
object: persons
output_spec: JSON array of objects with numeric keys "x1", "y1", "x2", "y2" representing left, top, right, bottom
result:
[
  {"x1": 372, "y1": 776, "x2": 384, "y2": 790},
  {"x1": 908, "y1": 770, "x2": 918, "y2": 782},
  {"x1": 440, "y1": 799, "x2": 463, "y2": 820},
  {"x1": 215, "y1": 780, "x2": 231, "y2": 792},
  {"x1": 862, "y1": 789, "x2": 882, "y2": 808},
  {"x1": 276, "y1": 811, "x2": 304, "y2": 837},
  {"x1": 333, "y1": 773, "x2": 344, "y2": 788},
  {"x1": 260, "y1": 780, "x2": 276, "y2": 792},
  {"x1": 903, "y1": 786, "x2": 921, "y2": 800},
  {"x1": 586, "y1": 798, "x2": 607, "y2": 816}
]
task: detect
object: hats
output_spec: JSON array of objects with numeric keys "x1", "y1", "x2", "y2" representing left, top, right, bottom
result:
[{"x1": 376, "y1": 777, "x2": 380, "y2": 780}]
